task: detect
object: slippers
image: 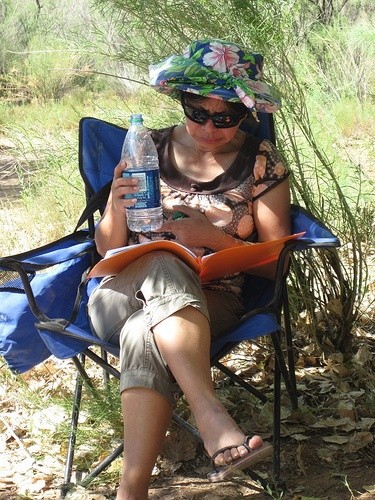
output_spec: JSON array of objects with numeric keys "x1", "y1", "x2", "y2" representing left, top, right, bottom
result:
[{"x1": 206, "y1": 435, "x2": 273, "y2": 483}]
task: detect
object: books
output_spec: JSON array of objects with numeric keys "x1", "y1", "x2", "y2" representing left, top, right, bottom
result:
[{"x1": 85, "y1": 232, "x2": 306, "y2": 285}]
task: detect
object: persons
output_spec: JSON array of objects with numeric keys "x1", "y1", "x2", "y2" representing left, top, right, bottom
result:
[{"x1": 87, "y1": 39, "x2": 293, "y2": 500}]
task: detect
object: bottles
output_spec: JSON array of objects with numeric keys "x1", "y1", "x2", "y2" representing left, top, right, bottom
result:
[{"x1": 120, "y1": 114, "x2": 163, "y2": 233}]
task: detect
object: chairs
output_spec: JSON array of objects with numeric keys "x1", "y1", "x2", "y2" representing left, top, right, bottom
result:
[{"x1": 0, "y1": 116, "x2": 341, "y2": 497}]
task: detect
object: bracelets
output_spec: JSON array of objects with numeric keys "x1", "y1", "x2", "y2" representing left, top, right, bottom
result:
[{"x1": 212, "y1": 231, "x2": 226, "y2": 250}]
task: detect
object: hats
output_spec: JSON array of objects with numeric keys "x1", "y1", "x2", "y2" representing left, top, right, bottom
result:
[{"x1": 149, "y1": 39, "x2": 282, "y2": 122}]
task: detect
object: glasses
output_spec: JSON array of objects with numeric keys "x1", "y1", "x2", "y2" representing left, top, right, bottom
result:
[{"x1": 180, "y1": 93, "x2": 249, "y2": 128}]
